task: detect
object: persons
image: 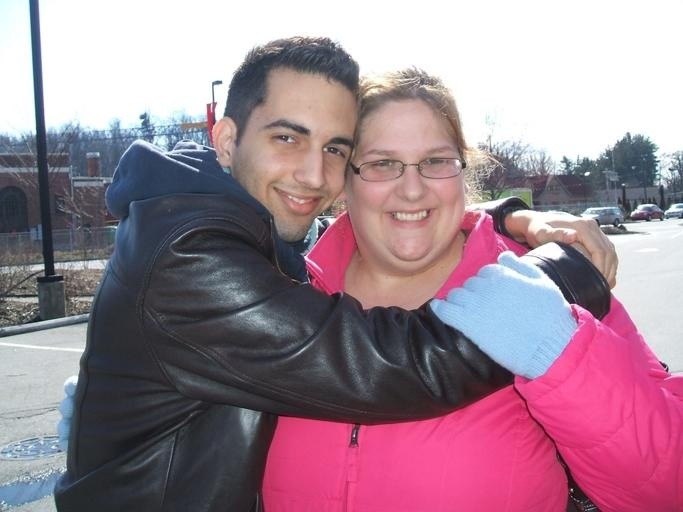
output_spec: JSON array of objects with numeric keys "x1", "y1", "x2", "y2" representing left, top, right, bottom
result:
[
  {"x1": 259, "y1": 64, "x2": 683, "y2": 511},
  {"x1": 52, "y1": 33, "x2": 618, "y2": 511}
]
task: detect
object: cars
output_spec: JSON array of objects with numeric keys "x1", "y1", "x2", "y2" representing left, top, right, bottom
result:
[
  {"x1": 628, "y1": 203, "x2": 666, "y2": 222},
  {"x1": 663, "y1": 203, "x2": 683, "y2": 219}
]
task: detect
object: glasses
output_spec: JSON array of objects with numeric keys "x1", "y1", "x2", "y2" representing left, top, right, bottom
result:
[{"x1": 349, "y1": 149, "x2": 466, "y2": 182}]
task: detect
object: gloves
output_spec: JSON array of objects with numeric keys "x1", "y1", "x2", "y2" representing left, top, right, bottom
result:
[
  {"x1": 57, "y1": 376, "x2": 78, "y2": 452},
  {"x1": 429, "y1": 251, "x2": 579, "y2": 380}
]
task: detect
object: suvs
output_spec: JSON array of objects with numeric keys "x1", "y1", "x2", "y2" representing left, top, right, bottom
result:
[{"x1": 577, "y1": 204, "x2": 625, "y2": 228}]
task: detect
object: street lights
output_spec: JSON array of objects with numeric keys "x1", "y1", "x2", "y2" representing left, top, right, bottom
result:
[
  {"x1": 572, "y1": 162, "x2": 587, "y2": 207},
  {"x1": 629, "y1": 165, "x2": 646, "y2": 204},
  {"x1": 210, "y1": 76, "x2": 223, "y2": 125}
]
